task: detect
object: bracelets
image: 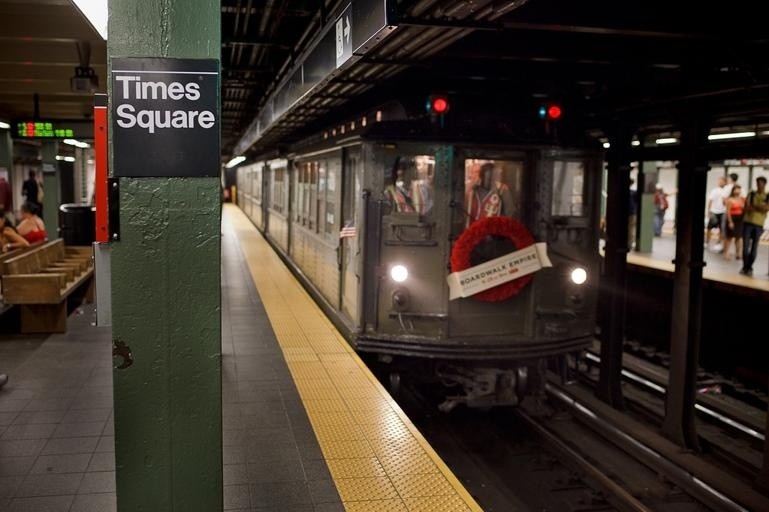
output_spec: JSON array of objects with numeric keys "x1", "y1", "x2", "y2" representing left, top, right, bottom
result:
[{"x1": 8, "y1": 243, "x2": 12, "y2": 248}]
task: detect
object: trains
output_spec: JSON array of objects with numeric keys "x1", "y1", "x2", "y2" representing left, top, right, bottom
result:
[{"x1": 237, "y1": 72, "x2": 607, "y2": 415}]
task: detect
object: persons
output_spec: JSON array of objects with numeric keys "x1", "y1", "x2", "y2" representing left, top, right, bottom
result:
[
  {"x1": 460, "y1": 164, "x2": 515, "y2": 231},
  {"x1": 654, "y1": 184, "x2": 668, "y2": 235},
  {"x1": 16, "y1": 202, "x2": 48, "y2": 243},
  {"x1": 0, "y1": 177, "x2": 10, "y2": 217},
  {"x1": 0, "y1": 210, "x2": 30, "y2": 253},
  {"x1": 628, "y1": 180, "x2": 637, "y2": 251},
  {"x1": 383, "y1": 166, "x2": 432, "y2": 218},
  {"x1": 21, "y1": 170, "x2": 43, "y2": 219},
  {"x1": 706, "y1": 174, "x2": 769, "y2": 277}
]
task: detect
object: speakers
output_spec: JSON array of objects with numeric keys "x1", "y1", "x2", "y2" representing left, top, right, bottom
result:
[{"x1": 71, "y1": 74, "x2": 100, "y2": 92}]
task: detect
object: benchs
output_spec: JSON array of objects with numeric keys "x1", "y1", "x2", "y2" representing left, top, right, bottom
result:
[{"x1": 0, "y1": 237, "x2": 96, "y2": 335}]
